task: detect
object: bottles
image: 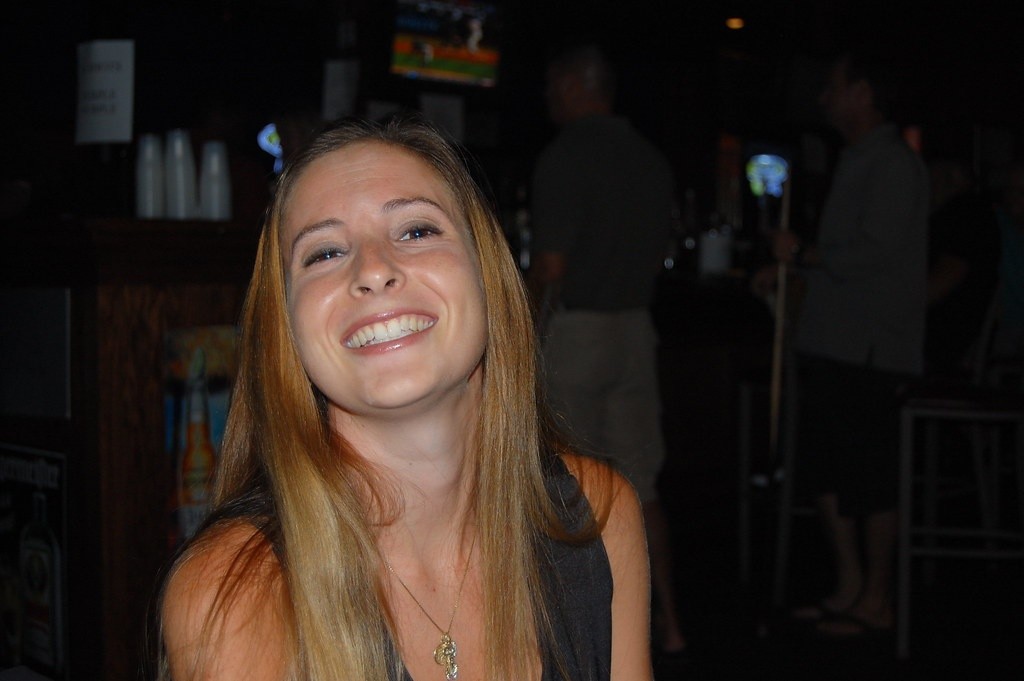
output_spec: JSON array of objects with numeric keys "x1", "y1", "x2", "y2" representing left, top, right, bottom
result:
[{"x1": 180, "y1": 381, "x2": 220, "y2": 537}]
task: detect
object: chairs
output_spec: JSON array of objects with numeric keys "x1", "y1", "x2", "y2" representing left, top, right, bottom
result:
[{"x1": 724, "y1": 262, "x2": 1023, "y2": 664}]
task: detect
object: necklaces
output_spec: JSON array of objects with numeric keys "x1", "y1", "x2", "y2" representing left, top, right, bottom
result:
[{"x1": 382, "y1": 532, "x2": 477, "y2": 680}]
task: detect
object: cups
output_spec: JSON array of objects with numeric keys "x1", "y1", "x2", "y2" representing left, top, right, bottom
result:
[
  {"x1": 134, "y1": 127, "x2": 232, "y2": 222},
  {"x1": 700, "y1": 233, "x2": 730, "y2": 274}
]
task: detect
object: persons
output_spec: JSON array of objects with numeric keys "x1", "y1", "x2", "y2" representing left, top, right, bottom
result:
[
  {"x1": 515, "y1": 39, "x2": 1023, "y2": 659},
  {"x1": 160, "y1": 113, "x2": 656, "y2": 681}
]
